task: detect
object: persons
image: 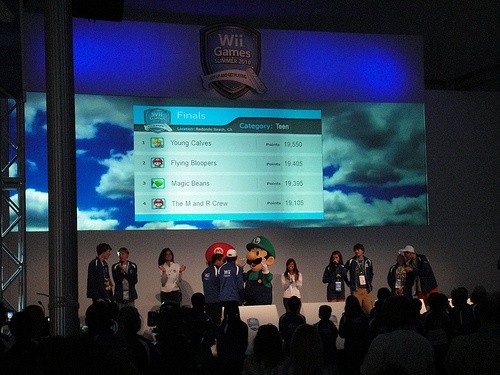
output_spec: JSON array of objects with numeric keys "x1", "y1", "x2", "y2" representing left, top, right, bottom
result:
[{"x1": 0, "y1": 243, "x2": 500, "y2": 375}]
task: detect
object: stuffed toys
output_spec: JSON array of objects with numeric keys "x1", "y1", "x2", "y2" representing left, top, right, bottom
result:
[
  {"x1": 204, "y1": 242, "x2": 235, "y2": 266},
  {"x1": 242, "y1": 236, "x2": 276, "y2": 306}
]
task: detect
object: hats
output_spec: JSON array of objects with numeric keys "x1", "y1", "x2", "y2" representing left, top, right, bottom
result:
[
  {"x1": 226, "y1": 248, "x2": 237, "y2": 258},
  {"x1": 400, "y1": 245, "x2": 414, "y2": 253}
]
